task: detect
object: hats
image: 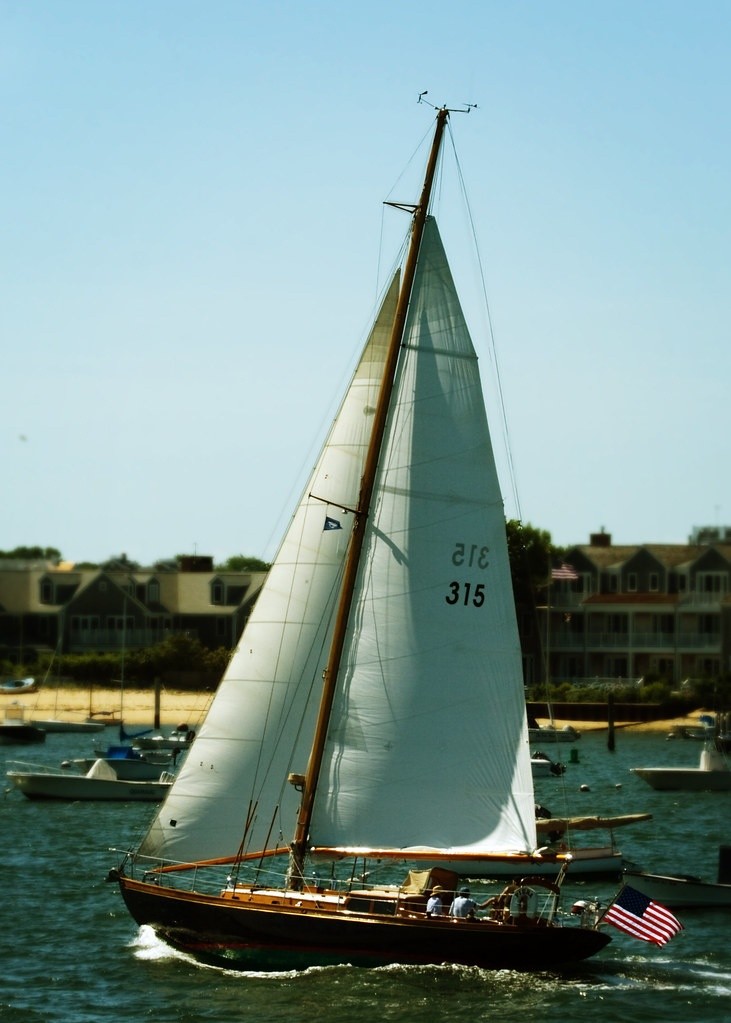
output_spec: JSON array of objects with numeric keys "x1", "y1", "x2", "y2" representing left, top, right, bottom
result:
[
  {"x1": 460, "y1": 887, "x2": 469, "y2": 892},
  {"x1": 429, "y1": 885, "x2": 444, "y2": 896}
]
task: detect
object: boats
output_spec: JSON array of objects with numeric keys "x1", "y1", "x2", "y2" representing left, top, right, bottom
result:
[
  {"x1": 0, "y1": 677, "x2": 198, "y2": 802},
  {"x1": 414, "y1": 709, "x2": 731, "y2": 909}
]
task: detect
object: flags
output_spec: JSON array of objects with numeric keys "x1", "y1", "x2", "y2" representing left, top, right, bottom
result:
[{"x1": 601, "y1": 884, "x2": 686, "y2": 951}]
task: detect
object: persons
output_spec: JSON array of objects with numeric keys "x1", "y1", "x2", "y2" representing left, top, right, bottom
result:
[
  {"x1": 426, "y1": 885, "x2": 448, "y2": 915},
  {"x1": 448, "y1": 886, "x2": 497, "y2": 918}
]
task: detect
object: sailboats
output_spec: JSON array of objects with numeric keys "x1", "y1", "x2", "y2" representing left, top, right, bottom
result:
[{"x1": 102, "y1": 88, "x2": 615, "y2": 979}]
